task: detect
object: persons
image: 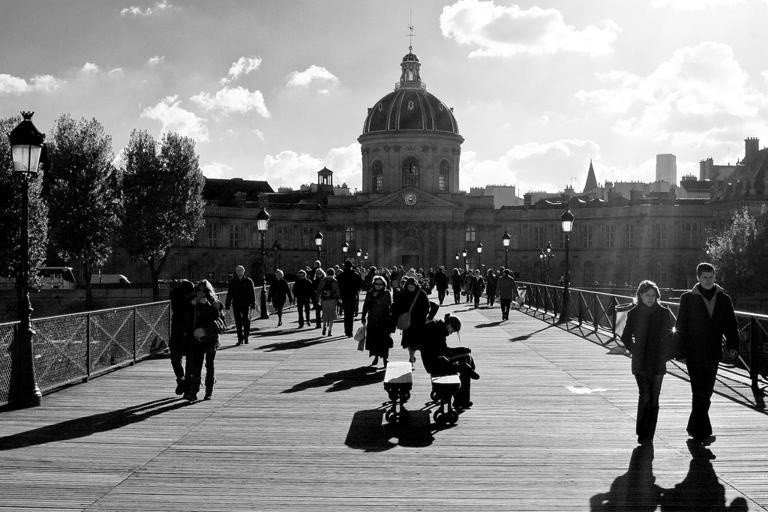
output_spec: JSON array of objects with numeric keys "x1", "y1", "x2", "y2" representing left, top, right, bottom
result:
[
  {"x1": 419, "y1": 313, "x2": 481, "y2": 408},
  {"x1": 224, "y1": 265, "x2": 256, "y2": 346},
  {"x1": 265, "y1": 256, "x2": 521, "y2": 368},
  {"x1": 171, "y1": 278, "x2": 224, "y2": 394},
  {"x1": 621, "y1": 280, "x2": 672, "y2": 445},
  {"x1": 672, "y1": 262, "x2": 740, "y2": 444},
  {"x1": 184, "y1": 278, "x2": 217, "y2": 402}
]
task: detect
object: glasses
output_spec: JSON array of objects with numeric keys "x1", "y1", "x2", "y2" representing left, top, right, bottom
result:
[{"x1": 374, "y1": 282, "x2": 383, "y2": 286}]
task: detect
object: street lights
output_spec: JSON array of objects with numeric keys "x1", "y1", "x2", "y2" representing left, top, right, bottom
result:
[
  {"x1": 314, "y1": 230, "x2": 370, "y2": 271},
  {"x1": 557, "y1": 207, "x2": 577, "y2": 324},
  {"x1": 4, "y1": 109, "x2": 54, "y2": 406},
  {"x1": 255, "y1": 204, "x2": 272, "y2": 321},
  {"x1": 538, "y1": 240, "x2": 556, "y2": 283},
  {"x1": 454, "y1": 230, "x2": 512, "y2": 271}
]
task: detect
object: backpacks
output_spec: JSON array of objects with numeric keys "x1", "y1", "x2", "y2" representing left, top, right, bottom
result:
[{"x1": 320, "y1": 277, "x2": 338, "y2": 302}]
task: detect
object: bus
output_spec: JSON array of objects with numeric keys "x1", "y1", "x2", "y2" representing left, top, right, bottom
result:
[{"x1": 1, "y1": 264, "x2": 78, "y2": 291}]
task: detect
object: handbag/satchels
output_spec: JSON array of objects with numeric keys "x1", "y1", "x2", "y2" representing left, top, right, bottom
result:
[
  {"x1": 353, "y1": 322, "x2": 367, "y2": 352},
  {"x1": 210, "y1": 318, "x2": 226, "y2": 334},
  {"x1": 397, "y1": 312, "x2": 411, "y2": 331}
]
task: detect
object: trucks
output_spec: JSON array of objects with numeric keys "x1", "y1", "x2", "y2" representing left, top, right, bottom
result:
[{"x1": 89, "y1": 273, "x2": 134, "y2": 285}]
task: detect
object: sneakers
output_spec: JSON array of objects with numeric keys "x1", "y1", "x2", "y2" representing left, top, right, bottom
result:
[
  {"x1": 440, "y1": 299, "x2": 510, "y2": 322},
  {"x1": 459, "y1": 362, "x2": 480, "y2": 380},
  {"x1": 452, "y1": 401, "x2": 474, "y2": 408},
  {"x1": 174, "y1": 377, "x2": 213, "y2": 402},
  {"x1": 236, "y1": 320, "x2": 353, "y2": 346},
  {"x1": 638, "y1": 432, "x2": 717, "y2": 448}
]
